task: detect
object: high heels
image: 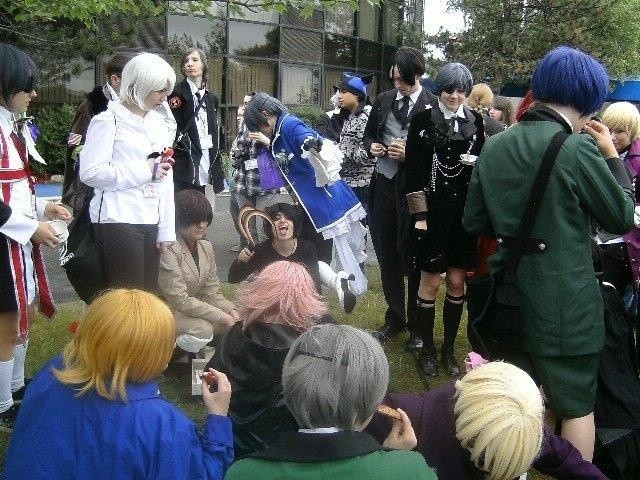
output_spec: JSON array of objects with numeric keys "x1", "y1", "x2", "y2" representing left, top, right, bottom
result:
[{"x1": 334, "y1": 270, "x2": 358, "y2": 314}]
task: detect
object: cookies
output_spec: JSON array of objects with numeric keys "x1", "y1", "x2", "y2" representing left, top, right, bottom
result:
[
  {"x1": 199, "y1": 371, "x2": 213, "y2": 385},
  {"x1": 377, "y1": 404, "x2": 402, "y2": 420}
]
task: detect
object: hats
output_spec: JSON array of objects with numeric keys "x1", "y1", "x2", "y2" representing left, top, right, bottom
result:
[{"x1": 339, "y1": 72, "x2": 375, "y2": 102}]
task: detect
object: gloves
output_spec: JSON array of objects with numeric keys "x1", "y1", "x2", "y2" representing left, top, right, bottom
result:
[{"x1": 410, "y1": 212, "x2": 431, "y2": 242}]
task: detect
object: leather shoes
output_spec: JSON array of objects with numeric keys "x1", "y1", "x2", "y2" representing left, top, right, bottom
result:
[
  {"x1": 418, "y1": 347, "x2": 439, "y2": 380},
  {"x1": 439, "y1": 350, "x2": 461, "y2": 378},
  {"x1": 405, "y1": 332, "x2": 424, "y2": 352},
  {"x1": 371, "y1": 323, "x2": 407, "y2": 342}
]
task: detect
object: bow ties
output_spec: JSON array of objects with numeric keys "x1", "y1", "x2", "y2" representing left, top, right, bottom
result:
[{"x1": 441, "y1": 114, "x2": 478, "y2": 139}]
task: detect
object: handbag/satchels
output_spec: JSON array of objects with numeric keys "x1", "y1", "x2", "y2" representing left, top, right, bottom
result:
[
  {"x1": 464, "y1": 266, "x2": 522, "y2": 363},
  {"x1": 57, "y1": 197, "x2": 107, "y2": 306}
]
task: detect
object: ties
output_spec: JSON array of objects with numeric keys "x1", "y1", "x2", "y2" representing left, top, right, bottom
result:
[
  {"x1": 398, "y1": 96, "x2": 410, "y2": 120},
  {"x1": 195, "y1": 91, "x2": 201, "y2": 104}
]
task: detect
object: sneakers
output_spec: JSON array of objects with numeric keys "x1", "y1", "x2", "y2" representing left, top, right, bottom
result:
[
  {"x1": 230, "y1": 240, "x2": 248, "y2": 252},
  {"x1": 12, "y1": 385, "x2": 27, "y2": 405},
  {"x1": 0, "y1": 404, "x2": 21, "y2": 435}
]
